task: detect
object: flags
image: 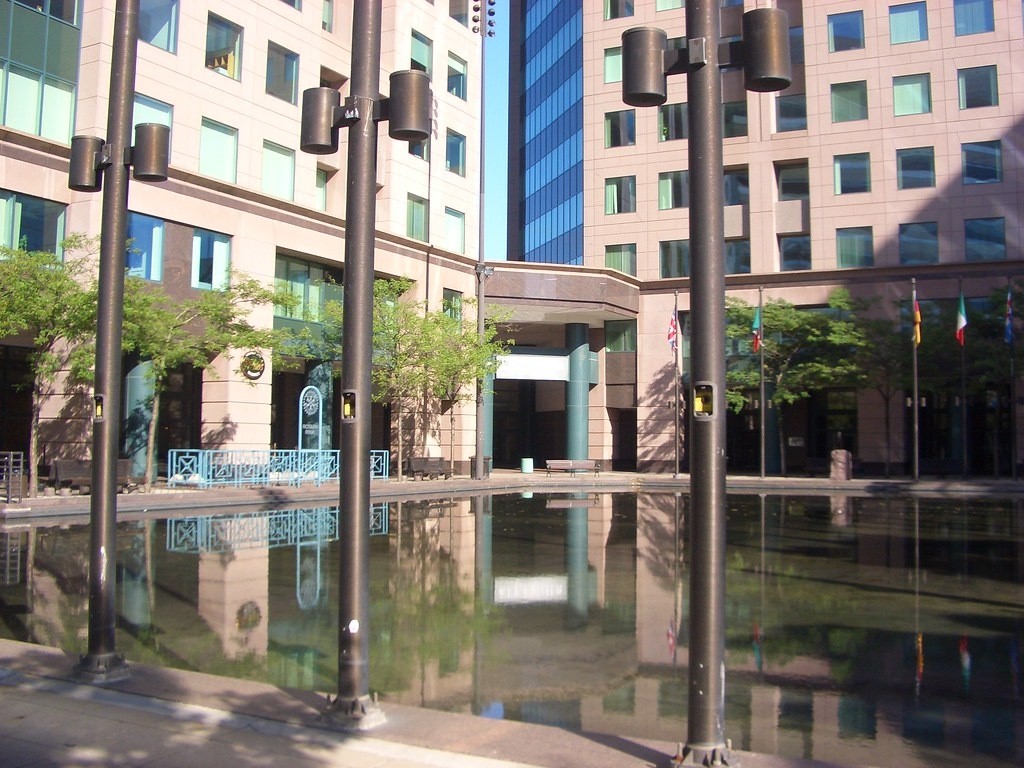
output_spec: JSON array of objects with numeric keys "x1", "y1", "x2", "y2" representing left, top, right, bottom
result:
[
  {"x1": 751, "y1": 304, "x2": 763, "y2": 352},
  {"x1": 954, "y1": 287, "x2": 967, "y2": 348},
  {"x1": 912, "y1": 289, "x2": 921, "y2": 348},
  {"x1": 1005, "y1": 282, "x2": 1014, "y2": 346},
  {"x1": 669, "y1": 301, "x2": 679, "y2": 354},
  {"x1": 666, "y1": 619, "x2": 976, "y2": 699}
]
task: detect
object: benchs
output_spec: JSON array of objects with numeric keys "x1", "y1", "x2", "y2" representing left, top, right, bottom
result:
[
  {"x1": 37, "y1": 459, "x2": 131, "y2": 494},
  {"x1": 40, "y1": 526, "x2": 147, "y2": 565},
  {"x1": 546, "y1": 459, "x2": 601, "y2": 478},
  {"x1": 394, "y1": 457, "x2": 458, "y2": 481},
  {"x1": 805, "y1": 457, "x2": 829, "y2": 478},
  {"x1": 545, "y1": 492, "x2": 599, "y2": 508},
  {"x1": 405, "y1": 501, "x2": 458, "y2": 519}
]
